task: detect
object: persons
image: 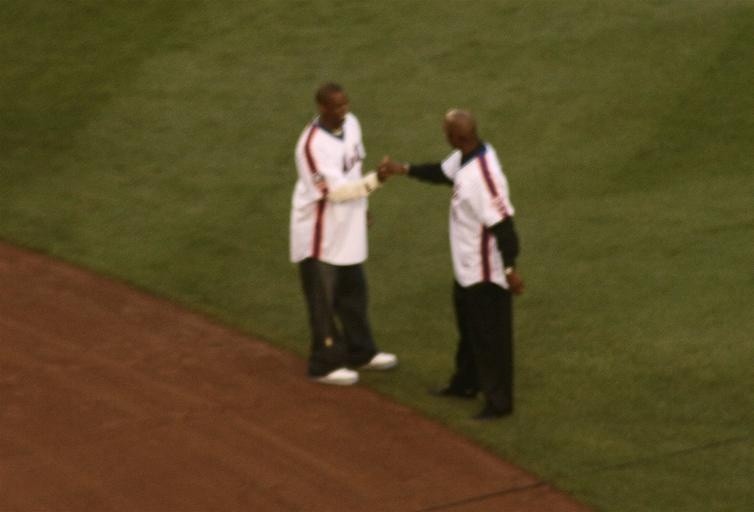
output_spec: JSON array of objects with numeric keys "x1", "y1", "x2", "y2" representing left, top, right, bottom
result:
[
  {"x1": 289, "y1": 83, "x2": 397, "y2": 388},
  {"x1": 375, "y1": 104, "x2": 524, "y2": 422}
]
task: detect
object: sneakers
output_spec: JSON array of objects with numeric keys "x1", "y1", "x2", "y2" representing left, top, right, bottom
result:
[
  {"x1": 359, "y1": 351, "x2": 397, "y2": 370},
  {"x1": 471, "y1": 404, "x2": 514, "y2": 420},
  {"x1": 430, "y1": 380, "x2": 479, "y2": 400},
  {"x1": 313, "y1": 367, "x2": 360, "y2": 387}
]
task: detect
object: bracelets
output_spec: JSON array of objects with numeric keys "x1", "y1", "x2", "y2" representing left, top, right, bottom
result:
[{"x1": 504, "y1": 267, "x2": 516, "y2": 274}]
